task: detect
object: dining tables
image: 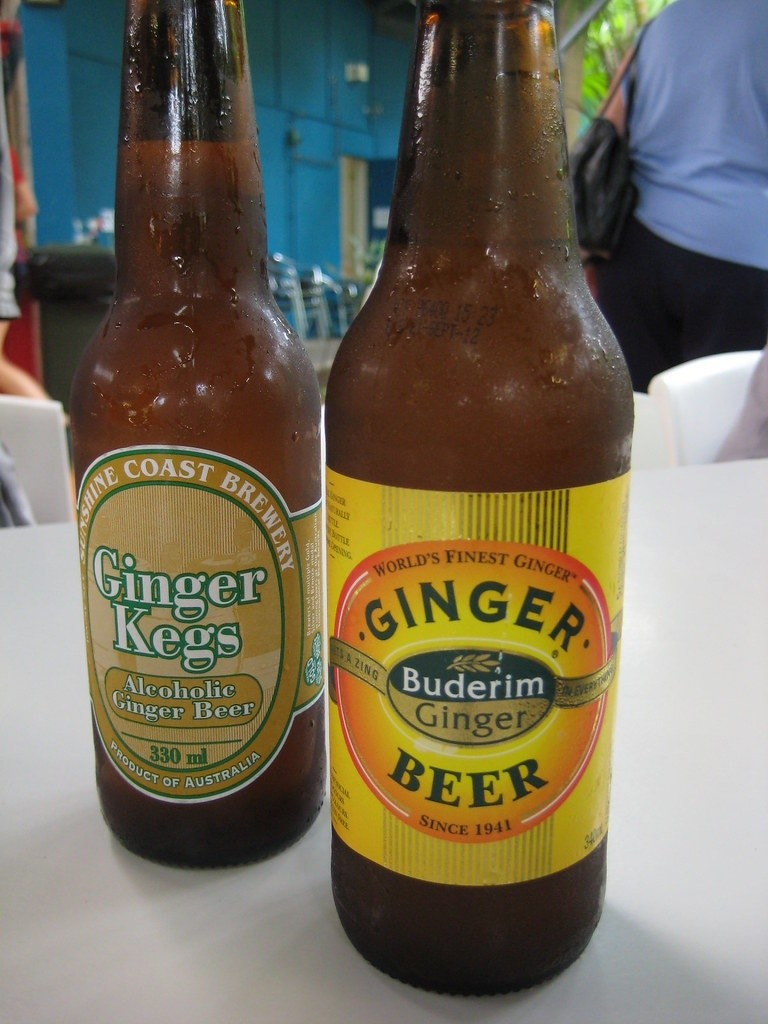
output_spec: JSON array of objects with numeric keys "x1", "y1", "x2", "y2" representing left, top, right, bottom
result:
[{"x1": 1, "y1": 454, "x2": 768, "y2": 1024}]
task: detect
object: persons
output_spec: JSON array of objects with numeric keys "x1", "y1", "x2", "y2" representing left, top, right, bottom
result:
[
  {"x1": 0, "y1": 0, "x2": 71, "y2": 529},
  {"x1": 598, "y1": 0, "x2": 768, "y2": 394}
]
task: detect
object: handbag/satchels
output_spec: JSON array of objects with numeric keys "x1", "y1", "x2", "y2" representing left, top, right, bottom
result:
[{"x1": 563, "y1": 19, "x2": 652, "y2": 261}]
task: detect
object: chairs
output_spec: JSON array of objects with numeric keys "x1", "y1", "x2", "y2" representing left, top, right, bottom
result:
[
  {"x1": 268, "y1": 250, "x2": 365, "y2": 342},
  {"x1": 648, "y1": 351, "x2": 767, "y2": 468}
]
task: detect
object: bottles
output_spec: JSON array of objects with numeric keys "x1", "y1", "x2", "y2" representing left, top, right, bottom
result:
[
  {"x1": 324, "y1": 0, "x2": 635, "y2": 999},
  {"x1": 65, "y1": 0, "x2": 326, "y2": 872}
]
